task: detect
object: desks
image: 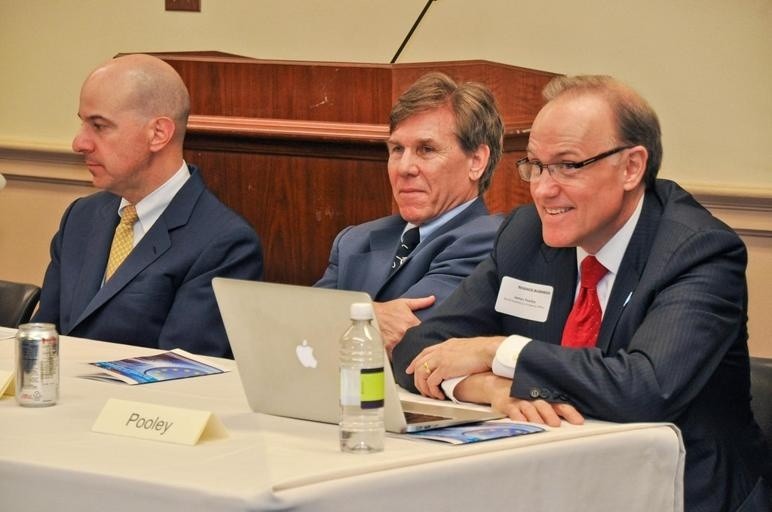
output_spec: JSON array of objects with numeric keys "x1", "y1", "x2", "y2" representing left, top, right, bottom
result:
[{"x1": 0, "y1": 322, "x2": 688, "y2": 512}]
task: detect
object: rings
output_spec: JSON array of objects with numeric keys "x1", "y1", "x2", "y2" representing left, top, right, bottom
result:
[{"x1": 424, "y1": 362, "x2": 432, "y2": 375}]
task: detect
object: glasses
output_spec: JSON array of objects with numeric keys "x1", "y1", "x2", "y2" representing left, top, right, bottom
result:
[{"x1": 514, "y1": 148, "x2": 623, "y2": 182}]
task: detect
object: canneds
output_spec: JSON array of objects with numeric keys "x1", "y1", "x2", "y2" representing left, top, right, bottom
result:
[{"x1": 15, "y1": 322, "x2": 60, "y2": 408}]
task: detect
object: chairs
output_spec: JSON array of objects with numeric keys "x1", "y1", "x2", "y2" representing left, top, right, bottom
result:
[{"x1": 0, "y1": 276, "x2": 41, "y2": 328}]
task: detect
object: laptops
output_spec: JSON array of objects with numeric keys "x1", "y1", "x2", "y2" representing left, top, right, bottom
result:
[{"x1": 211, "y1": 277, "x2": 509, "y2": 434}]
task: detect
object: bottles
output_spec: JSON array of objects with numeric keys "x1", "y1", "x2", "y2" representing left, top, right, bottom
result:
[{"x1": 336, "y1": 299, "x2": 388, "y2": 453}]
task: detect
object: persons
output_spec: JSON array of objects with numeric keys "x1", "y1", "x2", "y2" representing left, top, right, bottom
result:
[
  {"x1": 391, "y1": 72, "x2": 771, "y2": 510},
  {"x1": 24, "y1": 53, "x2": 264, "y2": 361},
  {"x1": 307, "y1": 72, "x2": 503, "y2": 360}
]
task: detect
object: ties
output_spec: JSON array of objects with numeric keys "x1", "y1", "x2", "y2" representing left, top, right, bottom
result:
[
  {"x1": 104, "y1": 203, "x2": 138, "y2": 285},
  {"x1": 561, "y1": 256, "x2": 609, "y2": 348},
  {"x1": 389, "y1": 226, "x2": 420, "y2": 276}
]
